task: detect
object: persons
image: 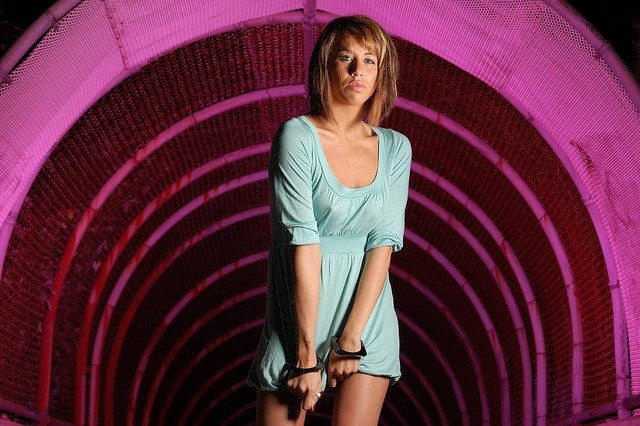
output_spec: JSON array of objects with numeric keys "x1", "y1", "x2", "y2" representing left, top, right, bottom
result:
[{"x1": 247, "y1": 14, "x2": 413, "y2": 426}]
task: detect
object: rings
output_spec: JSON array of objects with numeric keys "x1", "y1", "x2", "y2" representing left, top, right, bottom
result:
[{"x1": 315, "y1": 392, "x2": 322, "y2": 400}]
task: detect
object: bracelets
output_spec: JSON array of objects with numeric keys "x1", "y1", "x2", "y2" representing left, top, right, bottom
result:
[
  {"x1": 330, "y1": 335, "x2": 368, "y2": 357},
  {"x1": 286, "y1": 356, "x2": 324, "y2": 375}
]
